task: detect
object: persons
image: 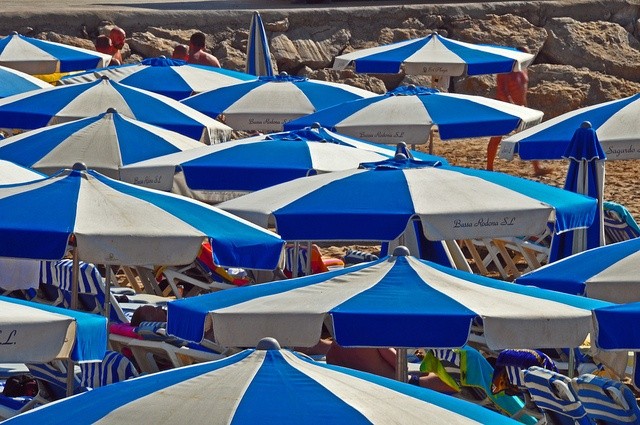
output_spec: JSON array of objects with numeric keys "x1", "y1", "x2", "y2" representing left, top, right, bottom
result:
[
  {"x1": 173, "y1": 45, "x2": 187, "y2": 61},
  {"x1": 326, "y1": 332, "x2": 459, "y2": 396},
  {"x1": 187, "y1": 33, "x2": 220, "y2": 68},
  {"x1": 488, "y1": 45, "x2": 549, "y2": 177},
  {"x1": 130, "y1": 304, "x2": 335, "y2": 354},
  {"x1": 96, "y1": 33, "x2": 109, "y2": 50},
  {"x1": 106, "y1": 27, "x2": 126, "y2": 66}
]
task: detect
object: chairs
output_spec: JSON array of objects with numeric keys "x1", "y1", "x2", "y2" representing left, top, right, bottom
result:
[
  {"x1": 571, "y1": 374, "x2": 639, "y2": 425},
  {"x1": 524, "y1": 366, "x2": 592, "y2": 425},
  {"x1": 0, "y1": 261, "x2": 222, "y2": 419},
  {"x1": 495, "y1": 348, "x2": 558, "y2": 403},
  {"x1": 455, "y1": 227, "x2": 551, "y2": 281},
  {"x1": 432, "y1": 331, "x2": 598, "y2": 409},
  {"x1": 158, "y1": 239, "x2": 381, "y2": 280},
  {"x1": 601, "y1": 198, "x2": 636, "y2": 244}
]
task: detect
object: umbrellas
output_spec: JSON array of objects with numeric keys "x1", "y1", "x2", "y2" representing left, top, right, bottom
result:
[
  {"x1": 0, "y1": 161, "x2": 287, "y2": 310},
  {"x1": 0, "y1": 107, "x2": 212, "y2": 192},
  {"x1": 546, "y1": 121, "x2": 606, "y2": 263},
  {"x1": 284, "y1": 83, "x2": 544, "y2": 153},
  {"x1": 58, "y1": 56, "x2": 259, "y2": 101},
  {"x1": 245, "y1": 10, "x2": 272, "y2": 76},
  {"x1": 0, "y1": 295, "x2": 109, "y2": 363},
  {"x1": 167, "y1": 244, "x2": 621, "y2": 384},
  {"x1": 0, "y1": 337, "x2": 523, "y2": 425},
  {"x1": 0, "y1": 66, "x2": 56, "y2": 97},
  {"x1": 499, "y1": 89, "x2": 640, "y2": 161},
  {"x1": 209, "y1": 142, "x2": 599, "y2": 246},
  {"x1": 333, "y1": 31, "x2": 534, "y2": 76},
  {"x1": 0, "y1": 31, "x2": 112, "y2": 74},
  {"x1": 513, "y1": 233, "x2": 640, "y2": 305},
  {"x1": 175, "y1": 71, "x2": 381, "y2": 131},
  {"x1": 0, "y1": 74, "x2": 233, "y2": 142},
  {"x1": 118, "y1": 121, "x2": 446, "y2": 191},
  {"x1": 0, "y1": 158, "x2": 50, "y2": 186}
]
